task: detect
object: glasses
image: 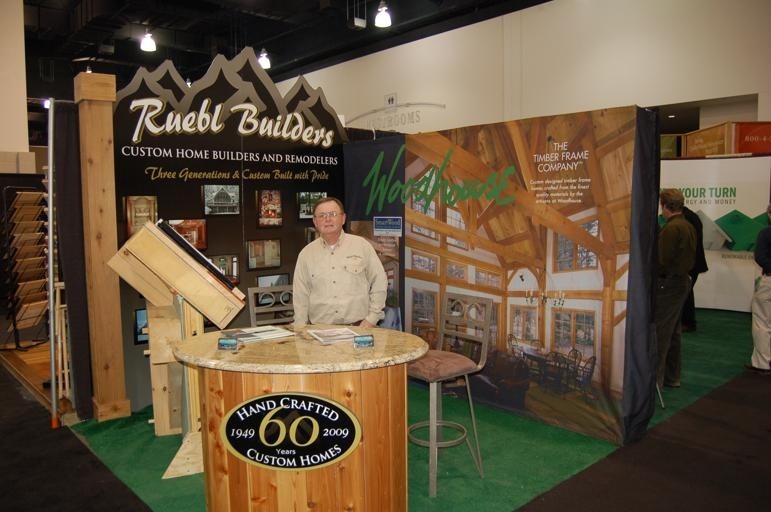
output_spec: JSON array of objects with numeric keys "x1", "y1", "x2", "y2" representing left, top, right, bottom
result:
[{"x1": 313, "y1": 212, "x2": 343, "y2": 219}]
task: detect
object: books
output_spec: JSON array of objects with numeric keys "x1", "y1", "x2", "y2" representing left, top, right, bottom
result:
[
  {"x1": 306, "y1": 328, "x2": 358, "y2": 346},
  {"x1": 220, "y1": 322, "x2": 296, "y2": 347}
]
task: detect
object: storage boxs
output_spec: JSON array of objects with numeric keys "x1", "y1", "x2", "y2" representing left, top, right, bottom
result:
[
  {"x1": 654, "y1": 133, "x2": 687, "y2": 162},
  {"x1": 680, "y1": 118, "x2": 771, "y2": 162}
]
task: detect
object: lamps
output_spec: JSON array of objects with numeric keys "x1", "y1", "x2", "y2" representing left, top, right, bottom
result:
[
  {"x1": 258, "y1": 52, "x2": 270, "y2": 77},
  {"x1": 372, "y1": 0, "x2": 395, "y2": 29},
  {"x1": 139, "y1": 22, "x2": 158, "y2": 56},
  {"x1": 521, "y1": 262, "x2": 567, "y2": 312}
]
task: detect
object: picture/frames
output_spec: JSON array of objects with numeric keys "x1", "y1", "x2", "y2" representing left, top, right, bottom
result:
[
  {"x1": 243, "y1": 238, "x2": 284, "y2": 272},
  {"x1": 132, "y1": 308, "x2": 152, "y2": 347},
  {"x1": 206, "y1": 254, "x2": 241, "y2": 290},
  {"x1": 253, "y1": 274, "x2": 290, "y2": 307}
]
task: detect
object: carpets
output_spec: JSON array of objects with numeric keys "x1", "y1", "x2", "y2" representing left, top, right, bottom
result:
[
  {"x1": 68, "y1": 351, "x2": 615, "y2": 512},
  {"x1": 649, "y1": 290, "x2": 760, "y2": 433},
  {"x1": 523, "y1": 376, "x2": 625, "y2": 437}
]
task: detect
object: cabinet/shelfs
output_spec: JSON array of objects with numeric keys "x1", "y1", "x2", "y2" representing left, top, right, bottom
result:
[{"x1": 1, "y1": 186, "x2": 50, "y2": 353}]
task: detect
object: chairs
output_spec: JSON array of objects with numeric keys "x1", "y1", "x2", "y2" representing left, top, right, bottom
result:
[
  {"x1": 505, "y1": 331, "x2": 601, "y2": 404},
  {"x1": 406, "y1": 287, "x2": 495, "y2": 500}
]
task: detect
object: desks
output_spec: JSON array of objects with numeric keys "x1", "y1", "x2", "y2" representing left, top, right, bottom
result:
[{"x1": 168, "y1": 320, "x2": 435, "y2": 512}]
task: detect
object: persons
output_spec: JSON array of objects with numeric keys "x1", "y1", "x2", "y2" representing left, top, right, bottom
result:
[
  {"x1": 743, "y1": 196, "x2": 770, "y2": 372},
  {"x1": 653, "y1": 186, "x2": 698, "y2": 393},
  {"x1": 679, "y1": 204, "x2": 710, "y2": 337},
  {"x1": 286, "y1": 197, "x2": 389, "y2": 328}
]
